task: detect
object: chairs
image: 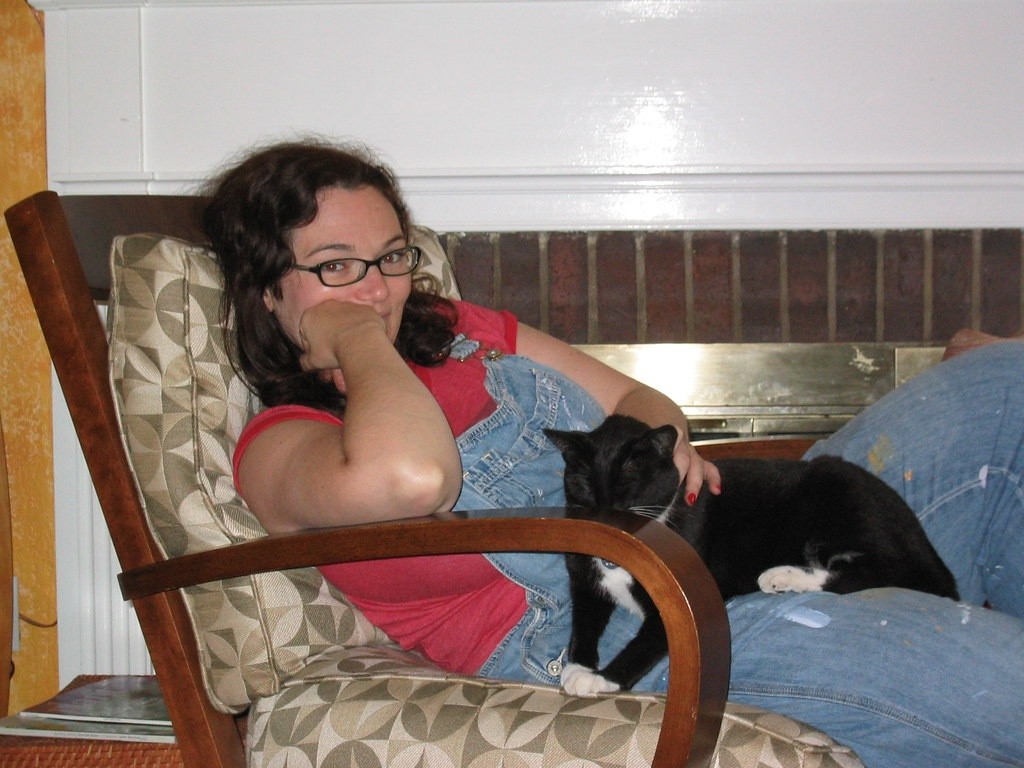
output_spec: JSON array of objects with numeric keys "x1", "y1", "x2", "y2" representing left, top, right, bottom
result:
[{"x1": 2, "y1": 190, "x2": 865, "y2": 768}]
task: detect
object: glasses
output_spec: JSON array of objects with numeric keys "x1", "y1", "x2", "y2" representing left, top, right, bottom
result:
[{"x1": 288, "y1": 245, "x2": 421, "y2": 287}]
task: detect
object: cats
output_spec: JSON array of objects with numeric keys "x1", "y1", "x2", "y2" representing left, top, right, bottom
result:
[{"x1": 537, "y1": 411, "x2": 961, "y2": 697}]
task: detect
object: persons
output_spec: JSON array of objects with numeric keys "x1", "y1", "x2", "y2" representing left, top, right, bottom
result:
[{"x1": 195, "y1": 144, "x2": 1024, "y2": 768}]
